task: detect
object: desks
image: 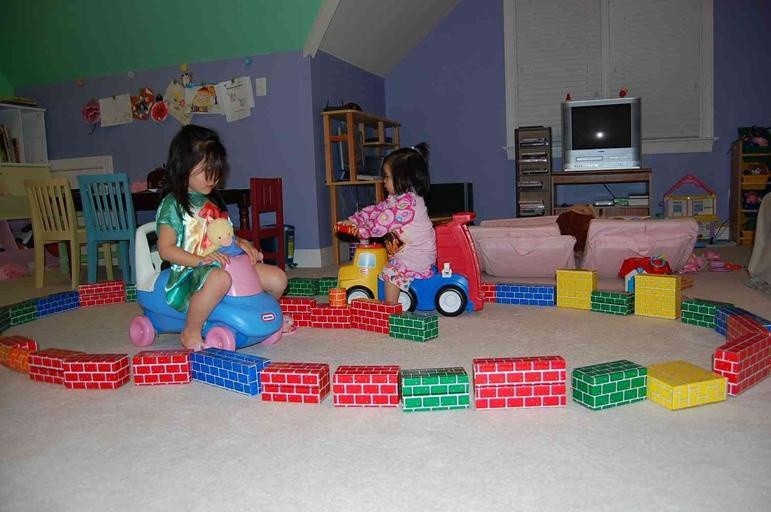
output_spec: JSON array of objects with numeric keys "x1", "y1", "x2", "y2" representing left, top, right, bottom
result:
[{"x1": 45, "y1": 185, "x2": 254, "y2": 279}]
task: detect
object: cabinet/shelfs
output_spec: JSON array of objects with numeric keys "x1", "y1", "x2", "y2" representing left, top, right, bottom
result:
[
  {"x1": 514, "y1": 124, "x2": 555, "y2": 219},
  {"x1": 729, "y1": 133, "x2": 771, "y2": 247},
  {"x1": 318, "y1": 110, "x2": 402, "y2": 268},
  {"x1": 0, "y1": 100, "x2": 51, "y2": 225},
  {"x1": 549, "y1": 167, "x2": 653, "y2": 226}
]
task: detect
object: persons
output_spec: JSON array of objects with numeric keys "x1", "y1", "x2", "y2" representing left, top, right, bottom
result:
[
  {"x1": 153, "y1": 123, "x2": 289, "y2": 354},
  {"x1": 337, "y1": 143, "x2": 441, "y2": 306}
]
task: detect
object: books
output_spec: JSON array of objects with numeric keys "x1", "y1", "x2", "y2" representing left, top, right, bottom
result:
[{"x1": 613, "y1": 193, "x2": 650, "y2": 206}]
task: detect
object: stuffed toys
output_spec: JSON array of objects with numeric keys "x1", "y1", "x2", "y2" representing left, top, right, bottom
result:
[{"x1": 201, "y1": 216, "x2": 255, "y2": 257}]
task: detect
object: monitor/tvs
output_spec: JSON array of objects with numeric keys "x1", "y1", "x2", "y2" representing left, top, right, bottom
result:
[{"x1": 562, "y1": 96, "x2": 641, "y2": 172}]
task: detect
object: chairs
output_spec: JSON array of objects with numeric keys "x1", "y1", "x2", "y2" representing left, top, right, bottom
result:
[
  {"x1": 75, "y1": 174, "x2": 163, "y2": 284},
  {"x1": 225, "y1": 176, "x2": 287, "y2": 275},
  {"x1": 22, "y1": 176, "x2": 114, "y2": 289}
]
